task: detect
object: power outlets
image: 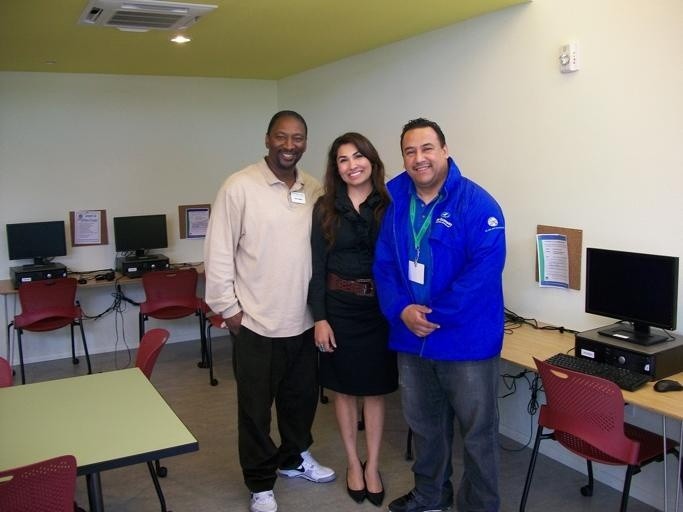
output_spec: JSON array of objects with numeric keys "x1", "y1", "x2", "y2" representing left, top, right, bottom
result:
[{"x1": 624, "y1": 399, "x2": 634, "y2": 417}]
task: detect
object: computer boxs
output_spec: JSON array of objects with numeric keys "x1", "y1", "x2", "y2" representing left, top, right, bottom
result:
[
  {"x1": 10, "y1": 262, "x2": 67, "y2": 288},
  {"x1": 115, "y1": 255, "x2": 169, "y2": 274},
  {"x1": 575, "y1": 322, "x2": 683, "y2": 380}
]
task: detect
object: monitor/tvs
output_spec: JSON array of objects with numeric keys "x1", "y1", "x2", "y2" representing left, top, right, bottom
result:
[
  {"x1": 6, "y1": 220, "x2": 66, "y2": 269},
  {"x1": 113, "y1": 214, "x2": 167, "y2": 260},
  {"x1": 584, "y1": 247, "x2": 679, "y2": 345}
]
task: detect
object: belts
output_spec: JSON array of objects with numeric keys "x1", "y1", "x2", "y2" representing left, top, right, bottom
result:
[{"x1": 327, "y1": 273, "x2": 375, "y2": 297}]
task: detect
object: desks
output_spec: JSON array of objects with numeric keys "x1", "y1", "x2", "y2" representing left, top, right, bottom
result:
[
  {"x1": 0, "y1": 259, "x2": 204, "y2": 372},
  {"x1": 500, "y1": 310, "x2": 683, "y2": 423},
  {"x1": 0, "y1": 363, "x2": 201, "y2": 510}
]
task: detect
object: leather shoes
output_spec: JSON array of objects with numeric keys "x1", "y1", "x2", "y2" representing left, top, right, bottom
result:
[
  {"x1": 347, "y1": 460, "x2": 367, "y2": 504},
  {"x1": 364, "y1": 461, "x2": 384, "y2": 507}
]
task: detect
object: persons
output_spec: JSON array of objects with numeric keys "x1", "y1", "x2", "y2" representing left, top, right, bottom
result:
[
  {"x1": 307, "y1": 132, "x2": 398, "y2": 508},
  {"x1": 373, "y1": 116, "x2": 506, "y2": 511},
  {"x1": 204, "y1": 111, "x2": 335, "y2": 512}
]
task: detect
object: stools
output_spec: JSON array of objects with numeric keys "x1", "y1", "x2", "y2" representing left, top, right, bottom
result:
[{"x1": 204, "y1": 312, "x2": 232, "y2": 386}]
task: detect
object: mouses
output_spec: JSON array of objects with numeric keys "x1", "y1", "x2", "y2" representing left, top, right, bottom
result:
[
  {"x1": 78, "y1": 279, "x2": 87, "y2": 284},
  {"x1": 654, "y1": 380, "x2": 683, "y2": 392}
]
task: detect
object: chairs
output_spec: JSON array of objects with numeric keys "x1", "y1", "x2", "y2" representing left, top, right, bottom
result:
[
  {"x1": 5, "y1": 276, "x2": 93, "y2": 385},
  {"x1": 0, "y1": 452, "x2": 83, "y2": 512},
  {"x1": 518, "y1": 354, "x2": 681, "y2": 511},
  {"x1": 137, "y1": 267, "x2": 212, "y2": 373},
  {"x1": 0, "y1": 357, "x2": 14, "y2": 388},
  {"x1": 133, "y1": 327, "x2": 170, "y2": 484}
]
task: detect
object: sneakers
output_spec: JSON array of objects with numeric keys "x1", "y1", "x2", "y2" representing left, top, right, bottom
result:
[
  {"x1": 387, "y1": 487, "x2": 454, "y2": 512},
  {"x1": 277, "y1": 450, "x2": 336, "y2": 483},
  {"x1": 249, "y1": 490, "x2": 278, "y2": 512}
]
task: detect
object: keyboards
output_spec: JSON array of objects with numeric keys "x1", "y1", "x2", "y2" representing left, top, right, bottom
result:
[
  {"x1": 125, "y1": 268, "x2": 179, "y2": 278},
  {"x1": 542, "y1": 353, "x2": 649, "y2": 392}
]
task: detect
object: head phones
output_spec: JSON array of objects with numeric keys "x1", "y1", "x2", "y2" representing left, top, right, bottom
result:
[{"x1": 95, "y1": 272, "x2": 115, "y2": 281}]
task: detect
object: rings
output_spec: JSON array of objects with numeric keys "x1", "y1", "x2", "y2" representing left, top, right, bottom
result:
[{"x1": 319, "y1": 344, "x2": 324, "y2": 352}]
